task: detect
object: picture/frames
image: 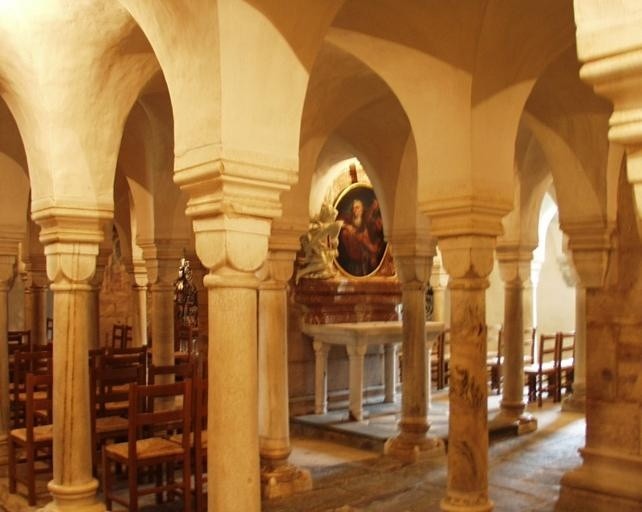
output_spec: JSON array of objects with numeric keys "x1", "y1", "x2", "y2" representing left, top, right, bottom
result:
[{"x1": 328, "y1": 181, "x2": 390, "y2": 280}]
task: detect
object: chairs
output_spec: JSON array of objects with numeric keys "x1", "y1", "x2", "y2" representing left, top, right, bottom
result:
[
  {"x1": 429, "y1": 326, "x2": 576, "y2": 409},
  {"x1": 5, "y1": 324, "x2": 208, "y2": 512}
]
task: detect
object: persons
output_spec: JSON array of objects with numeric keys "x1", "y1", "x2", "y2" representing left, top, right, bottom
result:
[{"x1": 339, "y1": 197, "x2": 369, "y2": 239}]
